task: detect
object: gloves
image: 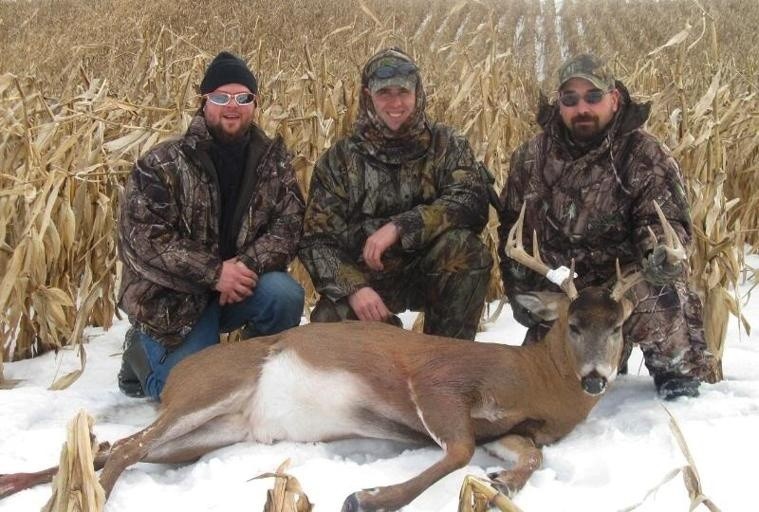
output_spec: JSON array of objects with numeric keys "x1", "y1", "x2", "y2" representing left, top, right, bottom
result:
[{"x1": 641, "y1": 247, "x2": 684, "y2": 287}]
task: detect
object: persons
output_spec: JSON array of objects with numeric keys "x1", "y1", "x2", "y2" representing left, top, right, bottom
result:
[
  {"x1": 298, "y1": 47, "x2": 495, "y2": 342},
  {"x1": 496, "y1": 52, "x2": 714, "y2": 403},
  {"x1": 116, "y1": 51, "x2": 307, "y2": 402}
]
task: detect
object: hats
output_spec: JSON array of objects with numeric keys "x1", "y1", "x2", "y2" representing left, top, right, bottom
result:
[
  {"x1": 558, "y1": 54, "x2": 614, "y2": 90},
  {"x1": 365, "y1": 64, "x2": 412, "y2": 87},
  {"x1": 199, "y1": 51, "x2": 258, "y2": 94},
  {"x1": 367, "y1": 56, "x2": 417, "y2": 95}
]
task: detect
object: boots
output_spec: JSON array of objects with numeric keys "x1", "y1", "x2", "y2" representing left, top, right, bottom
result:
[{"x1": 116, "y1": 329, "x2": 154, "y2": 399}]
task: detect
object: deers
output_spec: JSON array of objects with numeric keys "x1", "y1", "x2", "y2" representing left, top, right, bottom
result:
[{"x1": 0, "y1": 195, "x2": 688, "y2": 512}]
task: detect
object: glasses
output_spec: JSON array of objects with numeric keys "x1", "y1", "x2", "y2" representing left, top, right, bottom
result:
[
  {"x1": 202, "y1": 92, "x2": 258, "y2": 106},
  {"x1": 560, "y1": 90, "x2": 613, "y2": 106}
]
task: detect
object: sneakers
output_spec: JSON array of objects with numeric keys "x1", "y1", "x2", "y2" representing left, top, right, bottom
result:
[{"x1": 654, "y1": 372, "x2": 700, "y2": 400}]
